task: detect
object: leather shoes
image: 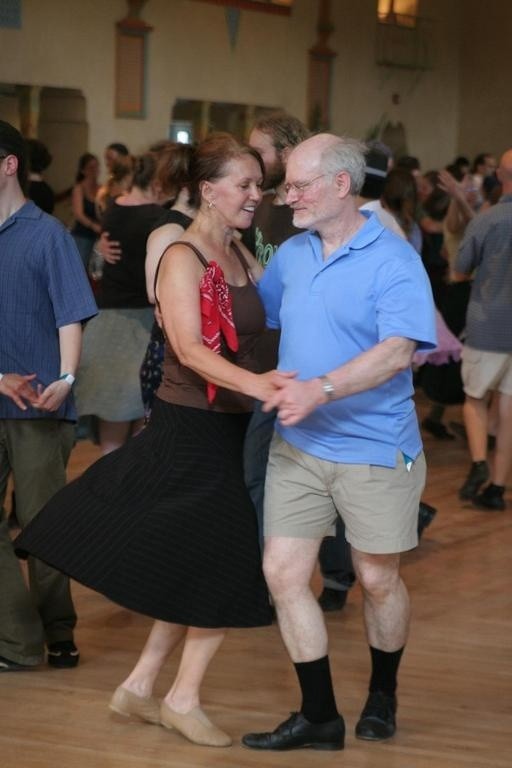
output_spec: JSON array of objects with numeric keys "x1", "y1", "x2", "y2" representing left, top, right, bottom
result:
[
  {"x1": 242, "y1": 711, "x2": 345, "y2": 751},
  {"x1": 449, "y1": 421, "x2": 496, "y2": 450},
  {"x1": 109, "y1": 687, "x2": 159, "y2": 723},
  {"x1": 0, "y1": 655, "x2": 38, "y2": 671},
  {"x1": 459, "y1": 466, "x2": 490, "y2": 502},
  {"x1": 418, "y1": 500, "x2": 436, "y2": 541},
  {"x1": 320, "y1": 573, "x2": 353, "y2": 610},
  {"x1": 420, "y1": 418, "x2": 454, "y2": 440},
  {"x1": 47, "y1": 639, "x2": 78, "y2": 668},
  {"x1": 354, "y1": 694, "x2": 398, "y2": 741},
  {"x1": 473, "y1": 494, "x2": 505, "y2": 511},
  {"x1": 159, "y1": 698, "x2": 232, "y2": 747}
]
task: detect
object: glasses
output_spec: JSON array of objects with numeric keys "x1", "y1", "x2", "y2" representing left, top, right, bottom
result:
[{"x1": 282, "y1": 171, "x2": 330, "y2": 195}]
